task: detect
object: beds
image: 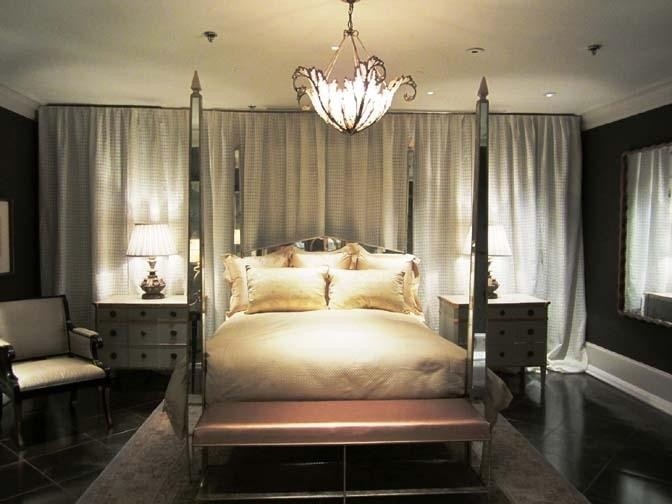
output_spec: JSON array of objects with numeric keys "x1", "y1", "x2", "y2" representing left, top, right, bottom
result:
[{"x1": 166, "y1": 72, "x2": 511, "y2": 478}]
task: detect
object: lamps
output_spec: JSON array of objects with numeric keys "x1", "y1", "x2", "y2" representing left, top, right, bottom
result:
[
  {"x1": 291, "y1": 0, "x2": 417, "y2": 136},
  {"x1": 460, "y1": 225, "x2": 511, "y2": 297},
  {"x1": 125, "y1": 223, "x2": 178, "y2": 299}
]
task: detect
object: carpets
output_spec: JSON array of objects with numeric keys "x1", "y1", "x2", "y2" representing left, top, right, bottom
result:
[{"x1": 69, "y1": 397, "x2": 590, "y2": 504}]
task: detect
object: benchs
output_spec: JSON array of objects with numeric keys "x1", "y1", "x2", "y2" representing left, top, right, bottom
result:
[{"x1": 191, "y1": 395, "x2": 492, "y2": 504}]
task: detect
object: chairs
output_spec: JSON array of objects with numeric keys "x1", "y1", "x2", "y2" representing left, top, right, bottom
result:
[{"x1": 0, "y1": 295, "x2": 115, "y2": 447}]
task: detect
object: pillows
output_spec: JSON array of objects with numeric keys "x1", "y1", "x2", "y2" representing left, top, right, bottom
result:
[
  {"x1": 327, "y1": 270, "x2": 410, "y2": 314},
  {"x1": 244, "y1": 264, "x2": 328, "y2": 315},
  {"x1": 355, "y1": 251, "x2": 425, "y2": 321},
  {"x1": 222, "y1": 247, "x2": 289, "y2": 317},
  {"x1": 289, "y1": 243, "x2": 356, "y2": 284}
]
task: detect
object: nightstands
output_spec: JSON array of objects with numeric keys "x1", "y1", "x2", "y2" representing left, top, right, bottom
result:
[
  {"x1": 438, "y1": 296, "x2": 550, "y2": 404},
  {"x1": 92, "y1": 295, "x2": 188, "y2": 376}
]
